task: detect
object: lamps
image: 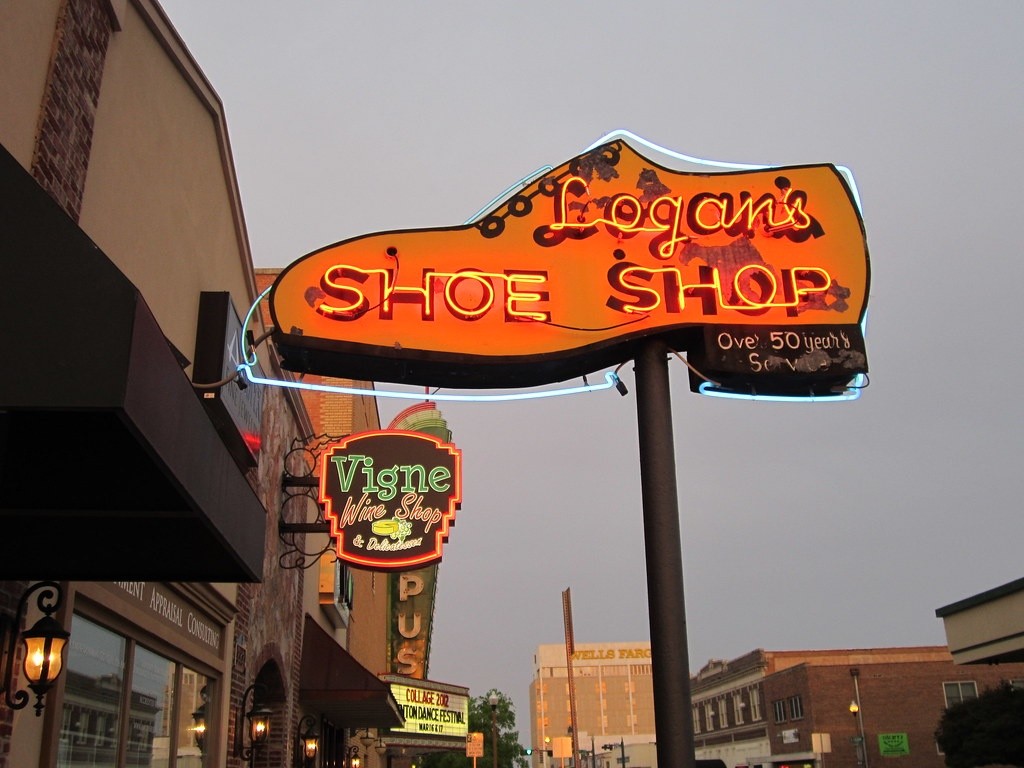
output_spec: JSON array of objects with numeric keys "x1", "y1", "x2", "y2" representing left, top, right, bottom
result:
[
  {"x1": 296, "y1": 714, "x2": 320, "y2": 767},
  {"x1": 3, "y1": 582, "x2": 71, "y2": 717},
  {"x1": 347, "y1": 746, "x2": 361, "y2": 768},
  {"x1": 241, "y1": 683, "x2": 273, "y2": 761}
]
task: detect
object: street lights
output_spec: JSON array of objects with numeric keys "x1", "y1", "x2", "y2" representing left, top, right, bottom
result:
[
  {"x1": 489, "y1": 691, "x2": 498, "y2": 768},
  {"x1": 545, "y1": 736, "x2": 550, "y2": 767},
  {"x1": 849, "y1": 700, "x2": 863, "y2": 768},
  {"x1": 191, "y1": 685, "x2": 208, "y2": 761}
]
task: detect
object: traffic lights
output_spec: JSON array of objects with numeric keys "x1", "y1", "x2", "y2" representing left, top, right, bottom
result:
[{"x1": 520, "y1": 750, "x2": 532, "y2": 755}]
